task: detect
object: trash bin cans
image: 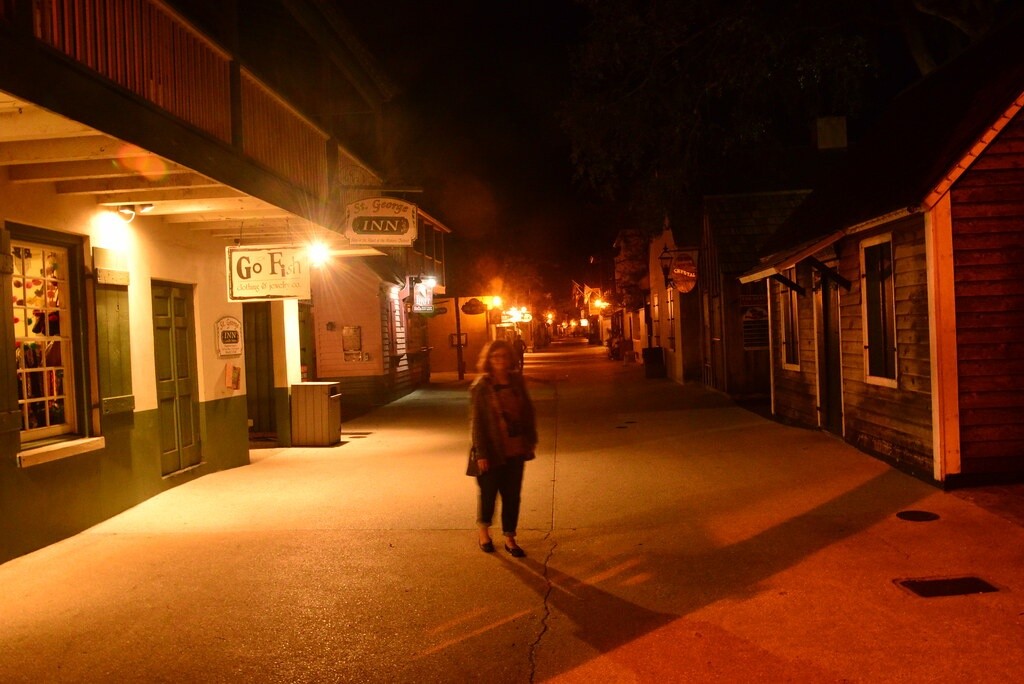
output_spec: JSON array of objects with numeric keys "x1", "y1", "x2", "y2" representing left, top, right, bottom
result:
[
  {"x1": 291, "y1": 381, "x2": 342, "y2": 446},
  {"x1": 641, "y1": 347, "x2": 665, "y2": 379}
]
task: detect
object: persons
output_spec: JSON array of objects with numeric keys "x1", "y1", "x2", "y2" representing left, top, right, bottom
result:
[{"x1": 469, "y1": 334, "x2": 538, "y2": 556}]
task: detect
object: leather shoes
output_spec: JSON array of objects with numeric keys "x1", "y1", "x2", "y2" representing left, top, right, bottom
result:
[
  {"x1": 479, "y1": 536, "x2": 494, "y2": 552},
  {"x1": 504, "y1": 541, "x2": 524, "y2": 557}
]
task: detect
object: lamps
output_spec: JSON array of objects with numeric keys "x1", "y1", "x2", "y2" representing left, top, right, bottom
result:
[
  {"x1": 413, "y1": 272, "x2": 425, "y2": 284},
  {"x1": 140, "y1": 203, "x2": 155, "y2": 215},
  {"x1": 116, "y1": 203, "x2": 137, "y2": 215}
]
